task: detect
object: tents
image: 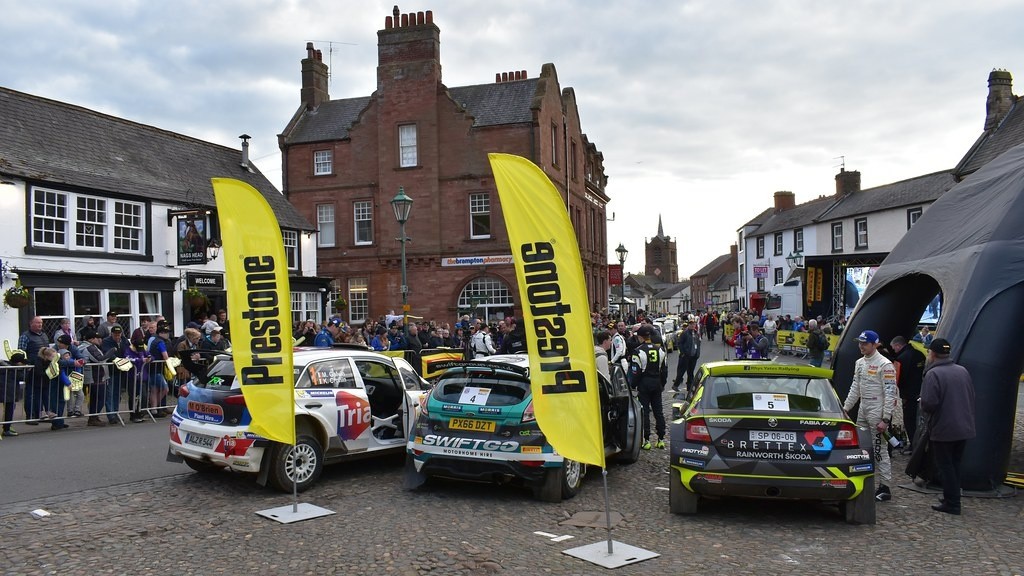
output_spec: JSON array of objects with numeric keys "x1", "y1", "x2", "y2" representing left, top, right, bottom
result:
[{"x1": 828, "y1": 138, "x2": 1024, "y2": 493}]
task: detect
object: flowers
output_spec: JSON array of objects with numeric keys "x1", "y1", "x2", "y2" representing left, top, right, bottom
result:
[
  {"x1": 181, "y1": 285, "x2": 211, "y2": 314},
  {"x1": 2, "y1": 284, "x2": 36, "y2": 315},
  {"x1": 334, "y1": 299, "x2": 348, "y2": 311}
]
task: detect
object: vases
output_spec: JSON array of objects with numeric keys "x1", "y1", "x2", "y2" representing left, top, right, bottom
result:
[
  {"x1": 189, "y1": 296, "x2": 205, "y2": 307},
  {"x1": 7, "y1": 295, "x2": 28, "y2": 308},
  {"x1": 335, "y1": 305, "x2": 346, "y2": 312}
]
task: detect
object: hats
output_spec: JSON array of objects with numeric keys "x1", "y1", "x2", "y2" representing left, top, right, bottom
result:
[
  {"x1": 331, "y1": 317, "x2": 347, "y2": 333},
  {"x1": 852, "y1": 330, "x2": 880, "y2": 344},
  {"x1": 11, "y1": 312, "x2": 201, "y2": 364},
  {"x1": 924, "y1": 338, "x2": 951, "y2": 354},
  {"x1": 635, "y1": 326, "x2": 650, "y2": 336}
]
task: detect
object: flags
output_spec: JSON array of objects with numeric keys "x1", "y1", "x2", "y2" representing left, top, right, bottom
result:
[
  {"x1": 209, "y1": 174, "x2": 297, "y2": 450},
  {"x1": 484, "y1": 149, "x2": 608, "y2": 469},
  {"x1": 607, "y1": 263, "x2": 623, "y2": 287}
]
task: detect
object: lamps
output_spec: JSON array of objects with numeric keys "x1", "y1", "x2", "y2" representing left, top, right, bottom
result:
[
  {"x1": 785, "y1": 252, "x2": 794, "y2": 266},
  {"x1": 793, "y1": 252, "x2": 804, "y2": 267},
  {"x1": 204, "y1": 238, "x2": 221, "y2": 264}
]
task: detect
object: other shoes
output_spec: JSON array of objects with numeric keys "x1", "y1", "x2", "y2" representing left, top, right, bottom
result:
[{"x1": 931, "y1": 498, "x2": 961, "y2": 515}]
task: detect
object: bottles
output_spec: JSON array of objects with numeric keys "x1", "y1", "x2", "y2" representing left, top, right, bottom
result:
[{"x1": 876, "y1": 423, "x2": 901, "y2": 449}]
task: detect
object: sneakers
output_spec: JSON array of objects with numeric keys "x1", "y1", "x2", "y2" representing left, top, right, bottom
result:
[
  {"x1": 0, "y1": 406, "x2": 172, "y2": 438},
  {"x1": 642, "y1": 439, "x2": 651, "y2": 450},
  {"x1": 875, "y1": 485, "x2": 891, "y2": 501},
  {"x1": 654, "y1": 439, "x2": 665, "y2": 449}
]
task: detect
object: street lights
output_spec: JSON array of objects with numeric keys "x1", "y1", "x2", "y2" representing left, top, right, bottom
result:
[
  {"x1": 390, "y1": 185, "x2": 414, "y2": 352},
  {"x1": 615, "y1": 243, "x2": 628, "y2": 323}
]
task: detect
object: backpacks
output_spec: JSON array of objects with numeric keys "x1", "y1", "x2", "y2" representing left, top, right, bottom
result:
[{"x1": 811, "y1": 330, "x2": 830, "y2": 352}]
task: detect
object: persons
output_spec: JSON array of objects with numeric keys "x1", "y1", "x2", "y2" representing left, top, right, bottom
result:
[{"x1": 2, "y1": 305, "x2": 981, "y2": 532}]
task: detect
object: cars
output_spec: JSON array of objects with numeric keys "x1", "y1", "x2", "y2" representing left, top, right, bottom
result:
[
  {"x1": 665, "y1": 360, "x2": 878, "y2": 528},
  {"x1": 633, "y1": 314, "x2": 685, "y2": 353},
  {"x1": 164, "y1": 347, "x2": 434, "y2": 495},
  {"x1": 404, "y1": 354, "x2": 644, "y2": 504}
]
